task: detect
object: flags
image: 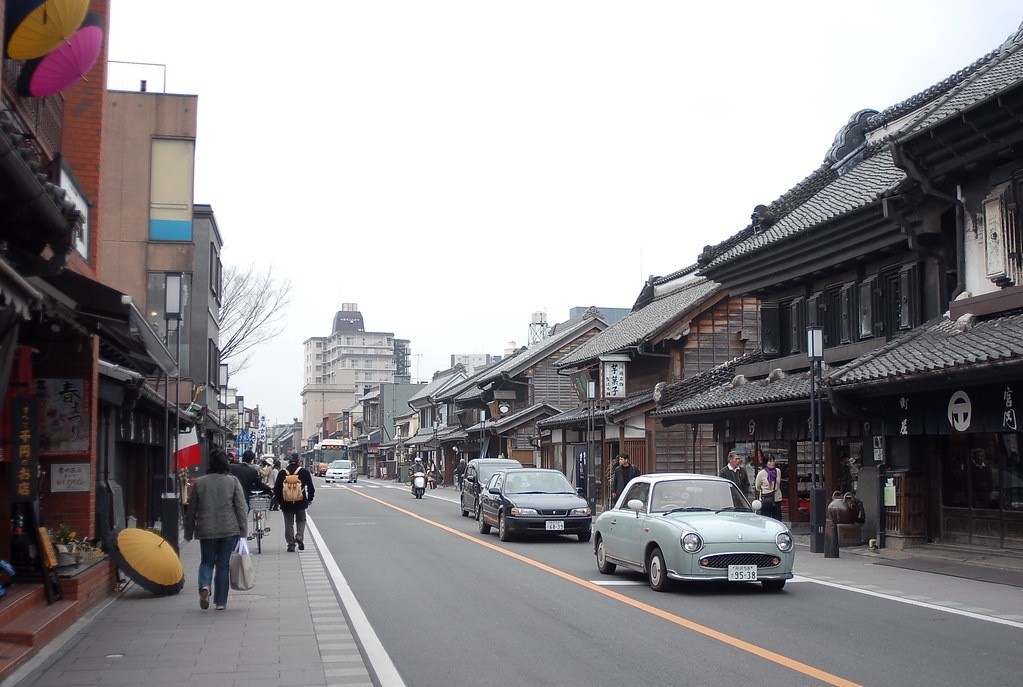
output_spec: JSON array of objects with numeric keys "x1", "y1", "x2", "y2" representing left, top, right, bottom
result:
[{"x1": 172, "y1": 425, "x2": 201, "y2": 472}]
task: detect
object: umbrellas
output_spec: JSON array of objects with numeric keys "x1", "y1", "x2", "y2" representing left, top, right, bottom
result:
[
  {"x1": 113, "y1": 527, "x2": 185, "y2": 596},
  {"x1": 8, "y1": 0, "x2": 90, "y2": 62},
  {"x1": 29, "y1": 26, "x2": 103, "y2": 97}
]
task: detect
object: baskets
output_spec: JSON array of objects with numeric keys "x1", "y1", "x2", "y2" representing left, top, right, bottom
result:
[{"x1": 249, "y1": 495, "x2": 271, "y2": 511}]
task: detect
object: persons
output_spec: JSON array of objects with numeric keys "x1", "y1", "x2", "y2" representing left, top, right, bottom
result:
[
  {"x1": 274, "y1": 453, "x2": 315, "y2": 552},
  {"x1": 719, "y1": 451, "x2": 750, "y2": 501},
  {"x1": 968, "y1": 448, "x2": 993, "y2": 506},
  {"x1": 755, "y1": 454, "x2": 783, "y2": 522},
  {"x1": 408, "y1": 458, "x2": 438, "y2": 491},
  {"x1": 183, "y1": 450, "x2": 248, "y2": 611},
  {"x1": 457, "y1": 459, "x2": 467, "y2": 493},
  {"x1": 611, "y1": 453, "x2": 641, "y2": 505},
  {"x1": 226, "y1": 450, "x2": 282, "y2": 514},
  {"x1": 501, "y1": 452, "x2": 508, "y2": 459}
]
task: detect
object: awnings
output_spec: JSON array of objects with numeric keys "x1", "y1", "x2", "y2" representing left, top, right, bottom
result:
[{"x1": 59, "y1": 266, "x2": 179, "y2": 379}]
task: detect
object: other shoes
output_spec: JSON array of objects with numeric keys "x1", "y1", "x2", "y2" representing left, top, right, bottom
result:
[
  {"x1": 216, "y1": 605, "x2": 224, "y2": 610},
  {"x1": 297, "y1": 538, "x2": 304, "y2": 550},
  {"x1": 287, "y1": 544, "x2": 295, "y2": 552},
  {"x1": 200, "y1": 586, "x2": 209, "y2": 609}
]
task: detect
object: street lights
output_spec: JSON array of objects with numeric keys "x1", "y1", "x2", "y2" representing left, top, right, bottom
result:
[
  {"x1": 433, "y1": 420, "x2": 438, "y2": 488},
  {"x1": 805, "y1": 325, "x2": 827, "y2": 553},
  {"x1": 161, "y1": 270, "x2": 183, "y2": 558},
  {"x1": 397, "y1": 428, "x2": 402, "y2": 483},
  {"x1": 238, "y1": 399, "x2": 244, "y2": 463},
  {"x1": 585, "y1": 379, "x2": 596, "y2": 516},
  {"x1": 217, "y1": 363, "x2": 229, "y2": 450},
  {"x1": 480, "y1": 409, "x2": 486, "y2": 458}
]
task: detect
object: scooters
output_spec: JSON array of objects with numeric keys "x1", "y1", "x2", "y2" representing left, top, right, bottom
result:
[{"x1": 408, "y1": 468, "x2": 430, "y2": 499}]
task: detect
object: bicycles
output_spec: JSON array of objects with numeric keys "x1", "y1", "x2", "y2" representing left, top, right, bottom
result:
[{"x1": 248, "y1": 490, "x2": 272, "y2": 555}]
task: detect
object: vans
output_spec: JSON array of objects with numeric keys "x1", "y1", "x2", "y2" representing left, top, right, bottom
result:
[{"x1": 460, "y1": 459, "x2": 524, "y2": 522}]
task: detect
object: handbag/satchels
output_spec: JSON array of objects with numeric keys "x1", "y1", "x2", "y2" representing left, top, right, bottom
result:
[
  {"x1": 761, "y1": 492, "x2": 774, "y2": 510},
  {"x1": 230, "y1": 537, "x2": 255, "y2": 590}
]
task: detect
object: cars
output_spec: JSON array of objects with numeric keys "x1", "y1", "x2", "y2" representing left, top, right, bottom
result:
[
  {"x1": 325, "y1": 459, "x2": 358, "y2": 483},
  {"x1": 478, "y1": 468, "x2": 592, "y2": 542},
  {"x1": 592, "y1": 473, "x2": 795, "y2": 594}
]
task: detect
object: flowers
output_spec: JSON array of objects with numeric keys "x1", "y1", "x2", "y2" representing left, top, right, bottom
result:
[{"x1": 50, "y1": 523, "x2": 77, "y2": 545}]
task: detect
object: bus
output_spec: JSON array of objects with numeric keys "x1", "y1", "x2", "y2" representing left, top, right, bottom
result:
[{"x1": 312, "y1": 439, "x2": 350, "y2": 477}]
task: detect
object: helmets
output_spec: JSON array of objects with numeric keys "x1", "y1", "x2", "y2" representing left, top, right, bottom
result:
[{"x1": 415, "y1": 457, "x2": 422, "y2": 465}]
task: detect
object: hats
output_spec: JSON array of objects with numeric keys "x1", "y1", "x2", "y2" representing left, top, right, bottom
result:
[
  {"x1": 621, "y1": 453, "x2": 628, "y2": 459},
  {"x1": 265, "y1": 458, "x2": 273, "y2": 466},
  {"x1": 289, "y1": 454, "x2": 298, "y2": 463}
]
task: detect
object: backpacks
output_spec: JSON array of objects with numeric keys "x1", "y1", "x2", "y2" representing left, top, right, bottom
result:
[{"x1": 283, "y1": 467, "x2": 304, "y2": 501}]
